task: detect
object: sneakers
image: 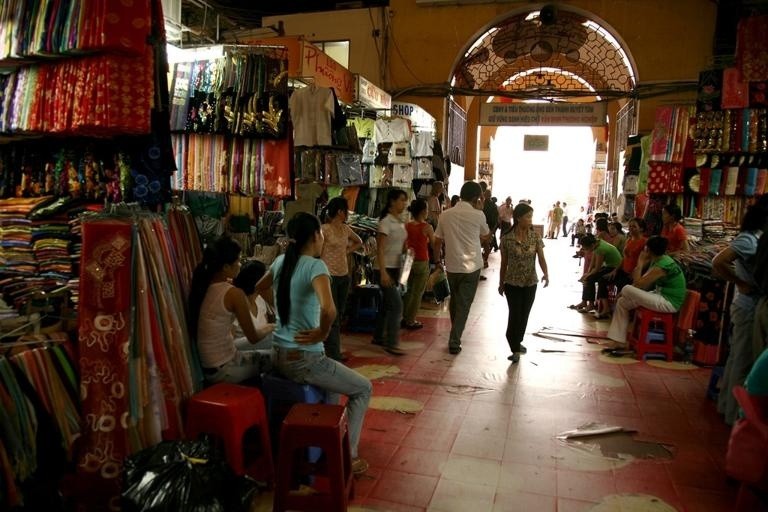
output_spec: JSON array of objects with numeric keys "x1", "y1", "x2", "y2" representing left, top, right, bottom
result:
[{"x1": 507, "y1": 343, "x2": 527, "y2": 361}]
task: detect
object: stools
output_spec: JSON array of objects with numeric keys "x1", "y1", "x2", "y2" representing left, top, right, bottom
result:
[
  {"x1": 274, "y1": 399, "x2": 355, "y2": 512},
  {"x1": 347, "y1": 283, "x2": 383, "y2": 332},
  {"x1": 183, "y1": 379, "x2": 278, "y2": 495},
  {"x1": 626, "y1": 306, "x2": 674, "y2": 364}
]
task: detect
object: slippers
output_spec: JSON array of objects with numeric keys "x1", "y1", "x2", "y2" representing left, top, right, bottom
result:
[
  {"x1": 400, "y1": 320, "x2": 422, "y2": 330},
  {"x1": 352, "y1": 458, "x2": 368, "y2": 475},
  {"x1": 570, "y1": 305, "x2": 610, "y2": 319},
  {"x1": 603, "y1": 346, "x2": 635, "y2": 358}
]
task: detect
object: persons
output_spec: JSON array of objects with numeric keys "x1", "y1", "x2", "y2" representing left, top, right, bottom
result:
[
  {"x1": 185, "y1": 237, "x2": 276, "y2": 384},
  {"x1": 316, "y1": 196, "x2": 363, "y2": 361},
  {"x1": 544, "y1": 201, "x2": 568, "y2": 240},
  {"x1": 710, "y1": 194, "x2": 768, "y2": 421},
  {"x1": 255, "y1": 212, "x2": 373, "y2": 473},
  {"x1": 432, "y1": 181, "x2": 492, "y2": 354},
  {"x1": 372, "y1": 189, "x2": 437, "y2": 354},
  {"x1": 427, "y1": 182, "x2": 514, "y2": 281},
  {"x1": 570, "y1": 203, "x2": 690, "y2": 356},
  {"x1": 498, "y1": 203, "x2": 548, "y2": 361}
]
